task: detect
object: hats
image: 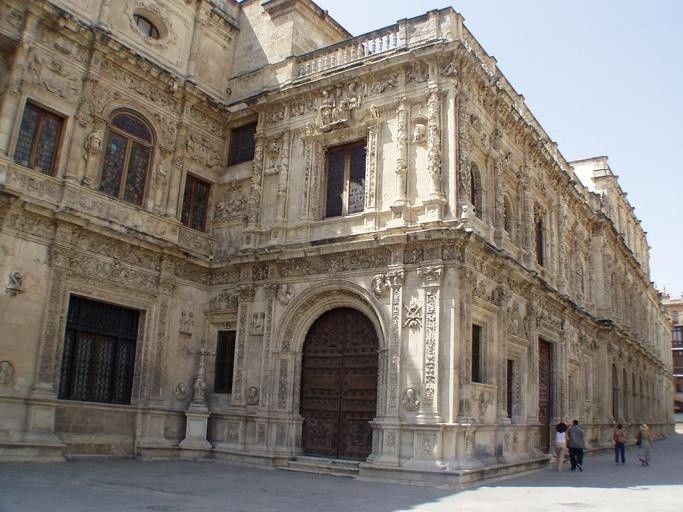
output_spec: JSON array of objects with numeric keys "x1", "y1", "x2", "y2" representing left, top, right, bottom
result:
[{"x1": 639, "y1": 423, "x2": 648, "y2": 431}]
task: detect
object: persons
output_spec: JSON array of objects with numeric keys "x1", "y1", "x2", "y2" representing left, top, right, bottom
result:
[
  {"x1": 613, "y1": 424, "x2": 626, "y2": 464},
  {"x1": 247, "y1": 388, "x2": 258, "y2": 404},
  {"x1": 567, "y1": 420, "x2": 585, "y2": 471},
  {"x1": 278, "y1": 284, "x2": 296, "y2": 306},
  {"x1": 555, "y1": 422, "x2": 570, "y2": 471},
  {"x1": 402, "y1": 389, "x2": 419, "y2": 410},
  {"x1": 636, "y1": 424, "x2": 654, "y2": 466},
  {"x1": 373, "y1": 274, "x2": 389, "y2": 297}
]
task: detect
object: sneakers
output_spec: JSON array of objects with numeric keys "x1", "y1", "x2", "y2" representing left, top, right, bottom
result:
[{"x1": 576, "y1": 462, "x2": 584, "y2": 472}]
